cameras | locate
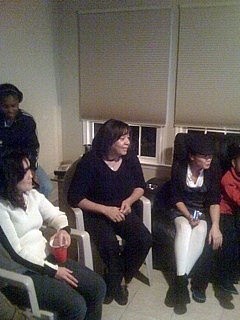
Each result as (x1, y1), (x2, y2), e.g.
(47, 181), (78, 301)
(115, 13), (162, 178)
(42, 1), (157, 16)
(193, 210), (202, 221)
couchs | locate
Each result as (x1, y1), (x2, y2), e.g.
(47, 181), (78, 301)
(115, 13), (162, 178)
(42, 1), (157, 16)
(144, 133), (240, 283)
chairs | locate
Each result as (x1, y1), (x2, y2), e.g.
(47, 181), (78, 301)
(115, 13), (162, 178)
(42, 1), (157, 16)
(62, 157), (154, 287)
(0, 226), (94, 320)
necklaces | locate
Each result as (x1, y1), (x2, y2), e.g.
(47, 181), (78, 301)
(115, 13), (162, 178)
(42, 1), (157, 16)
(103, 155), (122, 171)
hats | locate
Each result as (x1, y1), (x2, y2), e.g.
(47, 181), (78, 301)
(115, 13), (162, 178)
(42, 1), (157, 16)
(189, 134), (216, 155)
(227, 142), (240, 160)
(0, 83), (23, 103)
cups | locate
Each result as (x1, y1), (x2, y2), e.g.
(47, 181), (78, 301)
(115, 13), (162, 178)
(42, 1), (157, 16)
(49, 236), (70, 264)
(193, 210), (205, 227)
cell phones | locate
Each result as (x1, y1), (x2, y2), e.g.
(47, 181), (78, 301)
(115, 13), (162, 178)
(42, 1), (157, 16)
(32, 178), (40, 187)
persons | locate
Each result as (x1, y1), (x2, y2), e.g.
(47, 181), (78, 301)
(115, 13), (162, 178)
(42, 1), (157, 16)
(164, 135), (224, 316)
(67, 118), (152, 306)
(0, 150), (107, 320)
(0, 83), (53, 201)
(190, 145), (240, 303)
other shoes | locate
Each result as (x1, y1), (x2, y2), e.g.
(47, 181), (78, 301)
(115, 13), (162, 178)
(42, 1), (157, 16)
(174, 288), (189, 313)
(102, 277), (114, 304)
(114, 276), (128, 306)
(191, 284), (206, 303)
(164, 287), (175, 307)
(218, 281), (238, 294)
